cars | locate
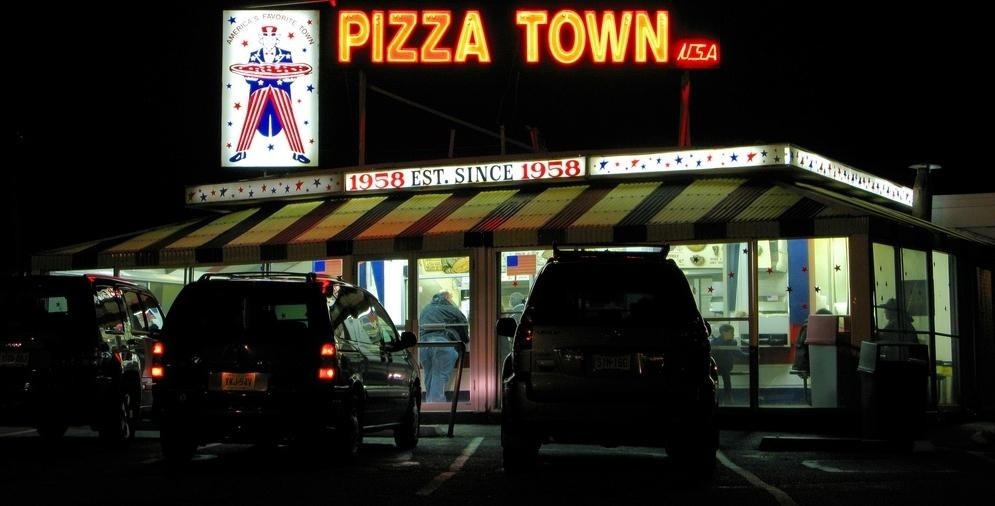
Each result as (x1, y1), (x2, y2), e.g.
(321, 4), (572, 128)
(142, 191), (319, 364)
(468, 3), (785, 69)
(150, 274), (422, 462)
(3, 273), (169, 443)
(493, 239), (722, 477)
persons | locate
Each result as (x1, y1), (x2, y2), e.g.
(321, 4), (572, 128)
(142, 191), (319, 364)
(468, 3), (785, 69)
(419, 291), (469, 403)
(793, 309), (850, 406)
(708, 324), (750, 405)
(507, 292), (525, 352)
(877, 296), (919, 357)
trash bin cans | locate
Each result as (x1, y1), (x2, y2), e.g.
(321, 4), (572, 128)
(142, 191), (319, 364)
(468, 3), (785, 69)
(803, 313), (929, 455)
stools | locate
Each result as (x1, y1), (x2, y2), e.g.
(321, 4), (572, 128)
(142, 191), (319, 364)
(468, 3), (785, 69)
(790, 370), (810, 403)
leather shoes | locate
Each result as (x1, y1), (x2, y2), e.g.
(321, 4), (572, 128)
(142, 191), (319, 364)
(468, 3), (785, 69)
(725, 391), (734, 405)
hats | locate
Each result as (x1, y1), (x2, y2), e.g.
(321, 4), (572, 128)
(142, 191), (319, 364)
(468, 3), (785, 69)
(510, 293), (524, 307)
(880, 298), (897, 309)
